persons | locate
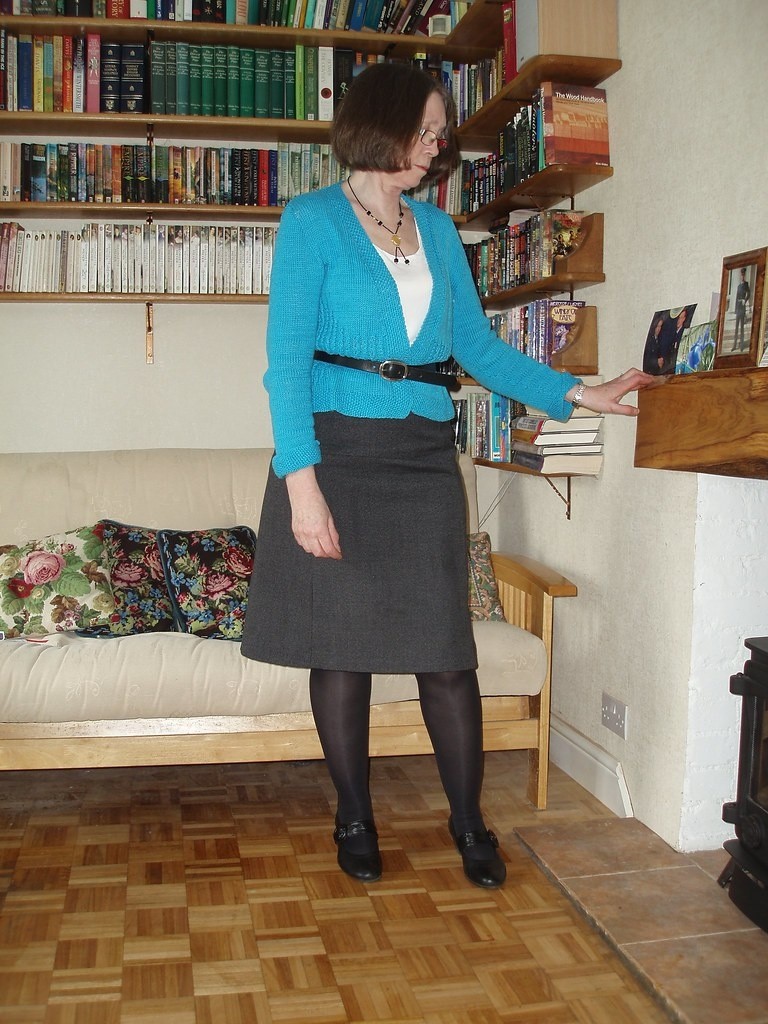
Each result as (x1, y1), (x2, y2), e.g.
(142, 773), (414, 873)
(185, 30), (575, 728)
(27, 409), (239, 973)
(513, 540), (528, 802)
(241, 62), (660, 890)
(646, 310), (687, 372)
(730, 268), (750, 351)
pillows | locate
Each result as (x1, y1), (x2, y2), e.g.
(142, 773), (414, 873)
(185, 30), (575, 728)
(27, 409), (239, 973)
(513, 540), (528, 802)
(0, 521), (121, 640)
(469, 532), (509, 622)
(154, 524), (257, 641)
(73, 519), (183, 640)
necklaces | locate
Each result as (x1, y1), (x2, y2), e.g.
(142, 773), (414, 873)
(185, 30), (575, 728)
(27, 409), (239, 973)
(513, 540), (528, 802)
(347, 175), (410, 266)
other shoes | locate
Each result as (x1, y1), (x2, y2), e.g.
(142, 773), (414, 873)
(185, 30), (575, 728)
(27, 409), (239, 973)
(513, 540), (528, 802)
(740, 347), (744, 351)
(731, 346), (736, 351)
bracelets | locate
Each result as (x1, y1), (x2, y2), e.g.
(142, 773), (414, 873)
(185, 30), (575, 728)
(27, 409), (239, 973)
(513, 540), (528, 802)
(571, 385), (587, 408)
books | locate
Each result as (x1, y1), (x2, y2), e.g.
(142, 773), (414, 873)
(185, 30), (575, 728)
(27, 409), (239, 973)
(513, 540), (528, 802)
(0, 0), (610, 294)
(439, 301), (605, 476)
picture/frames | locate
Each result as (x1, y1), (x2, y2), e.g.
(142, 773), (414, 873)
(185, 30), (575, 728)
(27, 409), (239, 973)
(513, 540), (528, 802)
(712, 246), (768, 370)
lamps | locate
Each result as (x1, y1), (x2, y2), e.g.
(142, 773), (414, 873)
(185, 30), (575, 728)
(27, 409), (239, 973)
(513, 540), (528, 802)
(428, 14), (451, 39)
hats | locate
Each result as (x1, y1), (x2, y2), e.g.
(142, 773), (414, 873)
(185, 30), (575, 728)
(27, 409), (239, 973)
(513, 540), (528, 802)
(741, 268), (746, 273)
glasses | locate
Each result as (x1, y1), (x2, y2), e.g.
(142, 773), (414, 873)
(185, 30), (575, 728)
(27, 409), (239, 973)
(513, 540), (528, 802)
(418, 127), (447, 150)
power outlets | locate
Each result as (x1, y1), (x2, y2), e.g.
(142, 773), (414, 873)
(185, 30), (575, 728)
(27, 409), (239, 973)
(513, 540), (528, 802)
(602, 692), (628, 741)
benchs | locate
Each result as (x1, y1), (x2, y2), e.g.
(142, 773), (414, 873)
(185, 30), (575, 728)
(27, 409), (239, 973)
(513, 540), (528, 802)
(0, 447), (578, 810)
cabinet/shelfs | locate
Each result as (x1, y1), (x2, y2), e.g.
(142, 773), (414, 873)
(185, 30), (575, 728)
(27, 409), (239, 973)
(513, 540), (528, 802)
(0, 0), (621, 520)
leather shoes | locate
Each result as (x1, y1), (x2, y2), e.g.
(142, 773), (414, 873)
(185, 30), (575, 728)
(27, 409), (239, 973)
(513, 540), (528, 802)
(333, 810), (380, 882)
(448, 813), (507, 889)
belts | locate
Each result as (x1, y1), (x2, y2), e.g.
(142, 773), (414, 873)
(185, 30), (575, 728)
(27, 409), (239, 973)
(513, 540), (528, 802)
(313, 350), (457, 388)
